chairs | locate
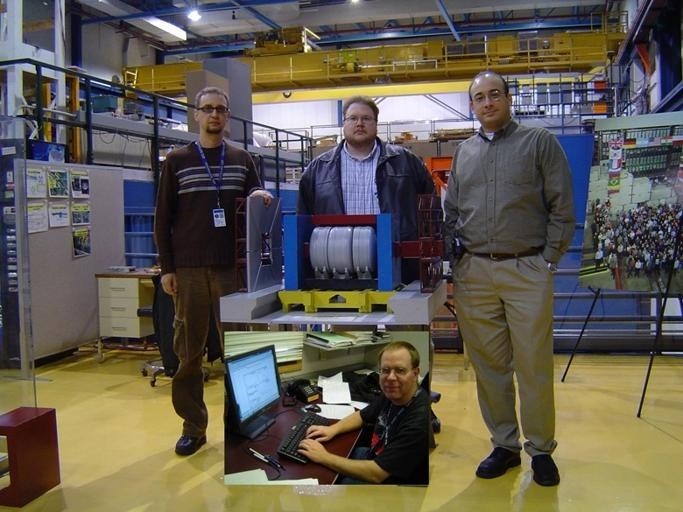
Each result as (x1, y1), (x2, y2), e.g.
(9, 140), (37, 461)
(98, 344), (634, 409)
(138, 276), (209, 387)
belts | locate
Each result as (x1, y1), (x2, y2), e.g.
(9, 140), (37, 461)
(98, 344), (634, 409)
(474, 250), (537, 261)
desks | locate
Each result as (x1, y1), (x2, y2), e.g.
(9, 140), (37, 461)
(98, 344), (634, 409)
(225, 381), (366, 484)
(95, 272), (163, 361)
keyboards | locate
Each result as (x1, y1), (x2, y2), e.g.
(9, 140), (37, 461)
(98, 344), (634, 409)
(277, 411), (330, 464)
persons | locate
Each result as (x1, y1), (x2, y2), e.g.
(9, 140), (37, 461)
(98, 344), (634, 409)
(298, 97), (445, 434)
(297, 341), (428, 485)
(154, 87), (275, 456)
(442, 71), (575, 485)
(590, 198), (683, 278)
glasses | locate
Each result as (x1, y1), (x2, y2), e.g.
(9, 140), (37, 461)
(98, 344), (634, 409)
(198, 104), (227, 115)
(377, 367), (411, 375)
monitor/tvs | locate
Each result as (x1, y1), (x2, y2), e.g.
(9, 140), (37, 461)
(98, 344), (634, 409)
(224, 344), (281, 441)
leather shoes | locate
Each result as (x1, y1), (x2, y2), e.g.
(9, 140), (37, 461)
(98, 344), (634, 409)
(530, 455), (560, 487)
(476, 448), (521, 478)
(175, 436), (206, 454)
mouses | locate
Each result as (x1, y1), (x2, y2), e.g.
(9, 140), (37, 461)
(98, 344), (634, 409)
(304, 404), (321, 413)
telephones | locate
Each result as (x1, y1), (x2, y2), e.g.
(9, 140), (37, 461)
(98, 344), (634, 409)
(287, 378), (319, 401)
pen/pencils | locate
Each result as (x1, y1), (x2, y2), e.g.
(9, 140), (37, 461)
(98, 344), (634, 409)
(248, 447), (287, 471)
